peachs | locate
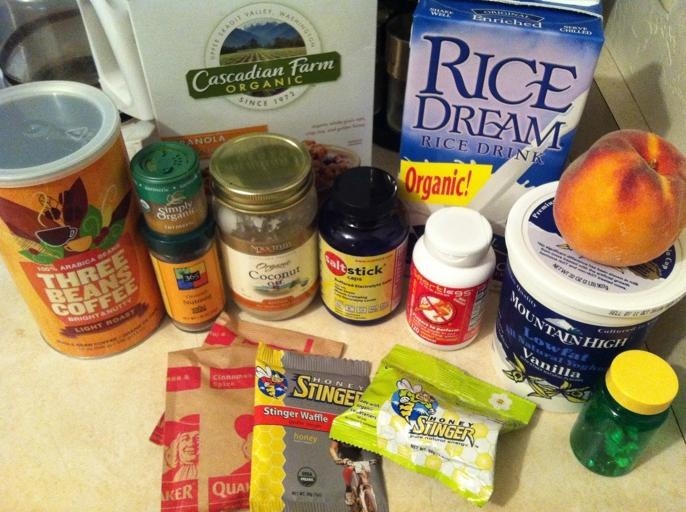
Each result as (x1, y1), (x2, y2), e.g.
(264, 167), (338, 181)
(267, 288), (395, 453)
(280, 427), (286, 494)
(553, 126), (686, 270)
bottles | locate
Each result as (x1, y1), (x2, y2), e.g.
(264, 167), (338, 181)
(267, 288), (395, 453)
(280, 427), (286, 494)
(316, 165), (411, 326)
(569, 349), (679, 478)
(404, 206), (496, 351)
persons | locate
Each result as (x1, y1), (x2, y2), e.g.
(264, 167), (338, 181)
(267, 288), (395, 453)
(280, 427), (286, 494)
(328, 440), (379, 512)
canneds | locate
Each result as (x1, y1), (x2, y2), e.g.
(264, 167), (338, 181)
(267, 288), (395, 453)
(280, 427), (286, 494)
(131, 140), (230, 332)
(208, 132), (320, 322)
